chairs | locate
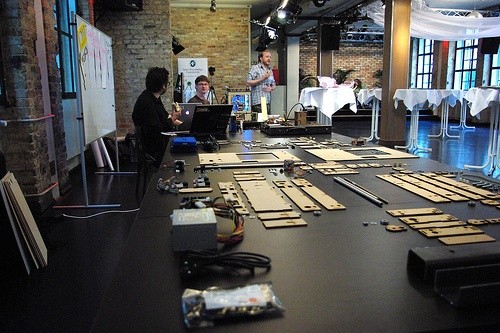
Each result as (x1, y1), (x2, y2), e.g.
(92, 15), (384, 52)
(125, 126), (162, 204)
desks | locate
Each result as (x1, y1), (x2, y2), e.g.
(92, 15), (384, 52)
(464, 86), (500, 177)
(89, 129), (500, 333)
(427, 89), (463, 139)
(393, 89), (436, 154)
(357, 88), (382, 142)
(450, 91), (481, 130)
(299, 86), (358, 127)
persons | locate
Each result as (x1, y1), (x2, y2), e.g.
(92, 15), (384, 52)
(352, 79), (367, 107)
(246, 50), (276, 114)
(132, 67), (182, 159)
(186, 74), (213, 105)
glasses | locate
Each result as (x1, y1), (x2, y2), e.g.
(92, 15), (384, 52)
(163, 81), (170, 85)
(197, 82), (208, 85)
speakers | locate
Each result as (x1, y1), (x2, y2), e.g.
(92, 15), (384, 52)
(321, 24), (340, 51)
(93, 0), (143, 11)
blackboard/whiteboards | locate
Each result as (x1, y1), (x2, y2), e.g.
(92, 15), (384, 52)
(76, 14), (116, 146)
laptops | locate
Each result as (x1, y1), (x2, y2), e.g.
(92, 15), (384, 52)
(175, 104), (234, 137)
